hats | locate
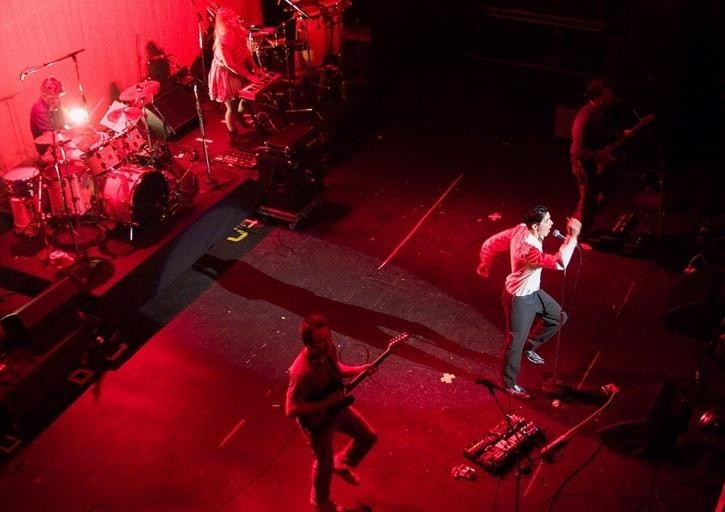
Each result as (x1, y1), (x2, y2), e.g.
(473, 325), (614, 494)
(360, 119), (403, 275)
(42, 78), (65, 98)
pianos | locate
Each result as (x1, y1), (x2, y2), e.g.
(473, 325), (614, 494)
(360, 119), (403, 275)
(239, 71), (283, 101)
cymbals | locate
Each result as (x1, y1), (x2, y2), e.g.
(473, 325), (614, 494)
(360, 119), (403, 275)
(35, 129), (72, 145)
(108, 107), (142, 122)
(119, 80), (160, 102)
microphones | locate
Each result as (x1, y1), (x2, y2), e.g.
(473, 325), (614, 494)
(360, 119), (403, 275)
(20, 72), (25, 80)
(474, 376), (511, 393)
(552, 229), (580, 249)
(48, 108), (55, 124)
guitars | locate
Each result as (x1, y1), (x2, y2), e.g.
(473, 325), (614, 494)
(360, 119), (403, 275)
(586, 115), (656, 175)
(297, 332), (409, 436)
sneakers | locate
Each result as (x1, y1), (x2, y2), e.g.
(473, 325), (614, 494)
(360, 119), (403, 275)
(318, 501), (338, 512)
(332, 466), (361, 486)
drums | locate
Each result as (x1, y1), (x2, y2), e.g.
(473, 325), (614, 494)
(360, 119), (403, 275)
(318, 0), (344, 56)
(79, 140), (122, 177)
(110, 124), (145, 161)
(295, 4), (327, 68)
(41, 160), (93, 218)
(97, 160), (169, 233)
(3, 166), (41, 199)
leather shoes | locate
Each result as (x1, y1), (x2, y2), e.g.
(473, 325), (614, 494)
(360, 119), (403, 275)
(524, 349), (545, 364)
(505, 384), (530, 399)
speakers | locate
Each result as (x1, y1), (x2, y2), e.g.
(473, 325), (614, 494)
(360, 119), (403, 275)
(662, 270), (721, 339)
(596, 382), (683, 458)
(140, 84), (205, 139)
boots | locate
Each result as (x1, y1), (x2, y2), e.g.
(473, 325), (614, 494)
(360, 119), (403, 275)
(228, 129), (250, 145)
(236, 112), (251, 127)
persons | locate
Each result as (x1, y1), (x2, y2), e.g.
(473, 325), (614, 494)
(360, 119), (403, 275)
(29, 77), (65, 156)
(207, 4), (265, 145)
(569, 78), (616, 250)
(147, 42), (171, 81)
(284, 314), (379, 512)
(476, 204), (582, 399)
